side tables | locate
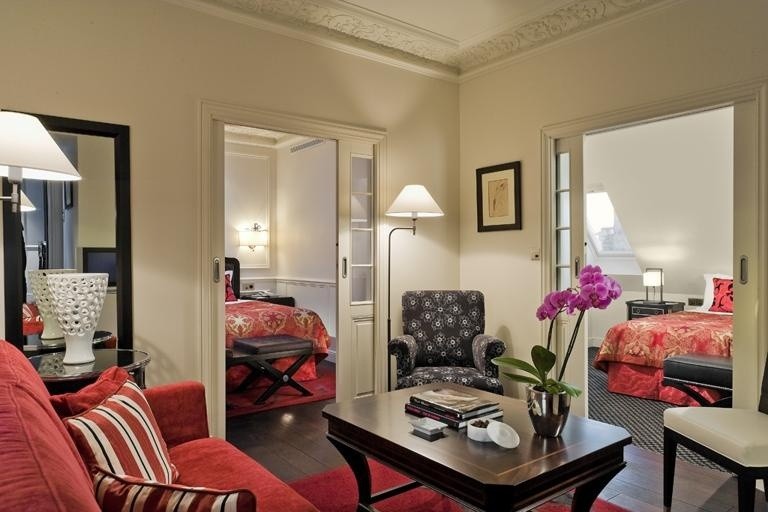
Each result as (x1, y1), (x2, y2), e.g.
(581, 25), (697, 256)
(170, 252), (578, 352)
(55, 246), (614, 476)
(28, 349), (151, 389)
(23, 331), (112, 357)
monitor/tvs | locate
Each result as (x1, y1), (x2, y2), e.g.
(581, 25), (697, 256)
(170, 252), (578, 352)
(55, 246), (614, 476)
(82, 247), (117, 293)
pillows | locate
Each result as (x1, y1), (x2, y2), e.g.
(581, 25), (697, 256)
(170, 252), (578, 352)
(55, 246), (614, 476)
(702, 273), (734, 315)
(49, 366), (256, 512)
(225, 270), (237, 302)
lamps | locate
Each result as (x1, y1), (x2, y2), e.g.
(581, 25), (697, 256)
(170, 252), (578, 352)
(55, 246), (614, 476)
(13, 184), (36, 212)
(643, 268), (665, 304)
(385, 185), (445, 392)
(0, 111), (82, 213)
(238, 223), (269, 257)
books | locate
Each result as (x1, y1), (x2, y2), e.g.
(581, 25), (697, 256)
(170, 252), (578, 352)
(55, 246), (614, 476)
(403, 401), (505, 431)
(410, 388), (501, 420)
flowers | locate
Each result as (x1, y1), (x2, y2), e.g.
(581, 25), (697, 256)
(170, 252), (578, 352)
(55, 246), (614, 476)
(491, 264), (623, 399)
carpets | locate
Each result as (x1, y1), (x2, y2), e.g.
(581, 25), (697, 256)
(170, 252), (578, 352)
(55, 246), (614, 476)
(288, 459), (632, 512)
(588, 346), (730, 473)
(226, 359), (336, 418)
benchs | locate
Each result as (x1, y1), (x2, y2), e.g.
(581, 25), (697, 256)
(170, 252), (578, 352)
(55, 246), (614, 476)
(661, 353), (733, 406)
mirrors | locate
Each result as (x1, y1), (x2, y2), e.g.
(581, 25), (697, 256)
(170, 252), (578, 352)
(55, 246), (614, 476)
(4, 110), (133, 370)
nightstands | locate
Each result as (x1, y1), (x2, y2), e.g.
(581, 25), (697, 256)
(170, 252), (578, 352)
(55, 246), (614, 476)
(243, 292), (295, 307)
(625, 300), (685, 320)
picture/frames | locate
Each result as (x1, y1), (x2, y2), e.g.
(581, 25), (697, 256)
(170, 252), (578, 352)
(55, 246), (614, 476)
(476, 161), (521, 232)
(63, 180), (73, 209)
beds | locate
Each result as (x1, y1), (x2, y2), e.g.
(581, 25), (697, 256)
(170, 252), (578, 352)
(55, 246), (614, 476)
(225, 257), (331, 393)
(606, 311), (733, 406)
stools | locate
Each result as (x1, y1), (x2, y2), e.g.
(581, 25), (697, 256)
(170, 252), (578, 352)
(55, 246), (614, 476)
(226, 335), (314, 404)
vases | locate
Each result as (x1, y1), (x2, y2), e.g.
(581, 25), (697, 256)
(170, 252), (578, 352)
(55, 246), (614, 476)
(526, 386), (571, 439)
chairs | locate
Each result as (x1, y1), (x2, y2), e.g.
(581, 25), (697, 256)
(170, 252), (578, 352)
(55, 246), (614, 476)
(388, 290), (506, 396)
(663, 353), (768, 512)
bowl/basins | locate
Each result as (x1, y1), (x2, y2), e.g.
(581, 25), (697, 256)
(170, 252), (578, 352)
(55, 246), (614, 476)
(467, 417), (520, 449)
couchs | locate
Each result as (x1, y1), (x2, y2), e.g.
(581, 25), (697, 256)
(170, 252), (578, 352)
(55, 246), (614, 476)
(0, 340), (320, 512)
